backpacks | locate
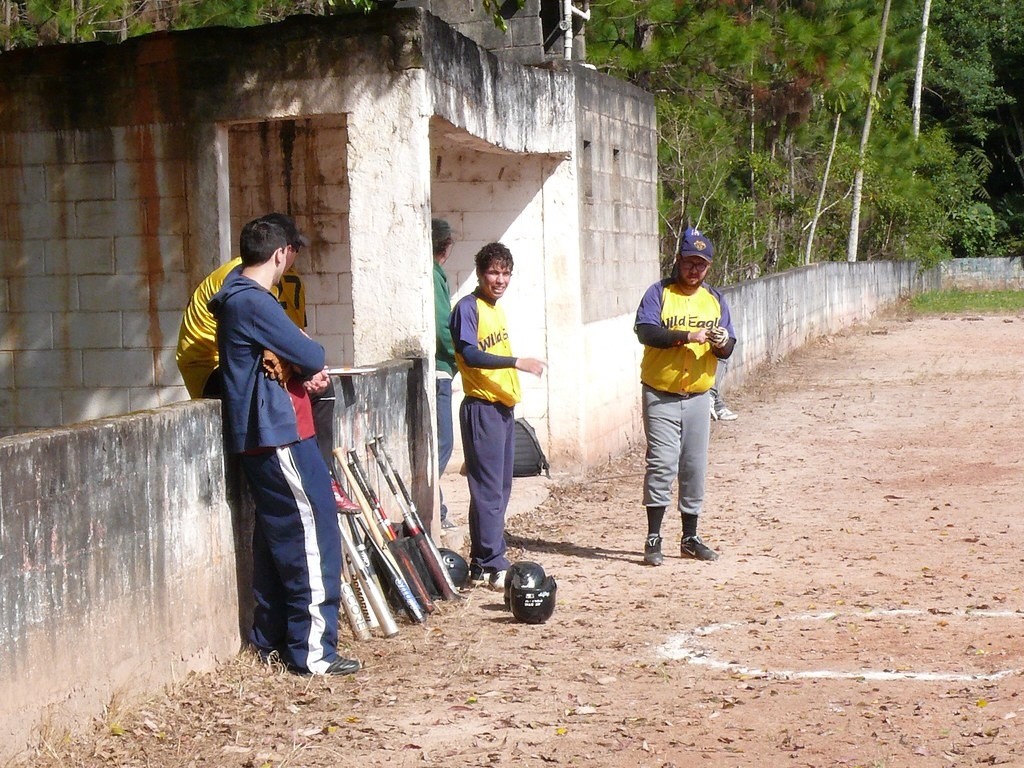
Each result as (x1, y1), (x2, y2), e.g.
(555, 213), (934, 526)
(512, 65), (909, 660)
(514, 416), (552, 480)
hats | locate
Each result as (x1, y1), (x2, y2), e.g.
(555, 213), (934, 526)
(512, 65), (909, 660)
(684, 226), (704, 234)
(680, 235), (714, 264)
(432, 218), (458, 243)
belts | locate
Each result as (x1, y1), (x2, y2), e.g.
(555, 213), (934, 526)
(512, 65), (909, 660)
(640, 381), (702, 399)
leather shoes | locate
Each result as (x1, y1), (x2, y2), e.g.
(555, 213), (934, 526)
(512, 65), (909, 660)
(331, 480), (362, 515)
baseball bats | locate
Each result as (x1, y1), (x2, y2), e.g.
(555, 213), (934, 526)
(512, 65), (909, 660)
(323, 432), (457, 643)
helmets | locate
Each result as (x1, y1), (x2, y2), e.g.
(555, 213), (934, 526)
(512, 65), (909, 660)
(503, 561), (557, 625)
(438, 547), (470, 587)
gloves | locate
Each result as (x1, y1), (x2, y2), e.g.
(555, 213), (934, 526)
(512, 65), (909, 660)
(705, 326), (730, 348)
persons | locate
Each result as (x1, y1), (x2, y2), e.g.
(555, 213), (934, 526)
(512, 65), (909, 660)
(634, 228), (737, 567)
(175, 214), (364, 517)
(449, 242), (549, 592)
(209, 220), (362, 674)
(432, 217), (459, 530)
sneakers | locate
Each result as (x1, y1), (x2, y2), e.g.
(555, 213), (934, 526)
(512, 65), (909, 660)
(680, 537), (720, 561)
(258, 650), (283, 664)
(644, 537), (664, 567)
(471, 570), (506, 593)
(325, 654), (361, 676)
(710, 393), (739, 422)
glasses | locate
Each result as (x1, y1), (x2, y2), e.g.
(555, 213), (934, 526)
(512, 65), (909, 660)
(680, 258), (709, 272)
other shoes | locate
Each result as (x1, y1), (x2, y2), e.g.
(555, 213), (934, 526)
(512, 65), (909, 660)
(440, 518), (457, 538)
